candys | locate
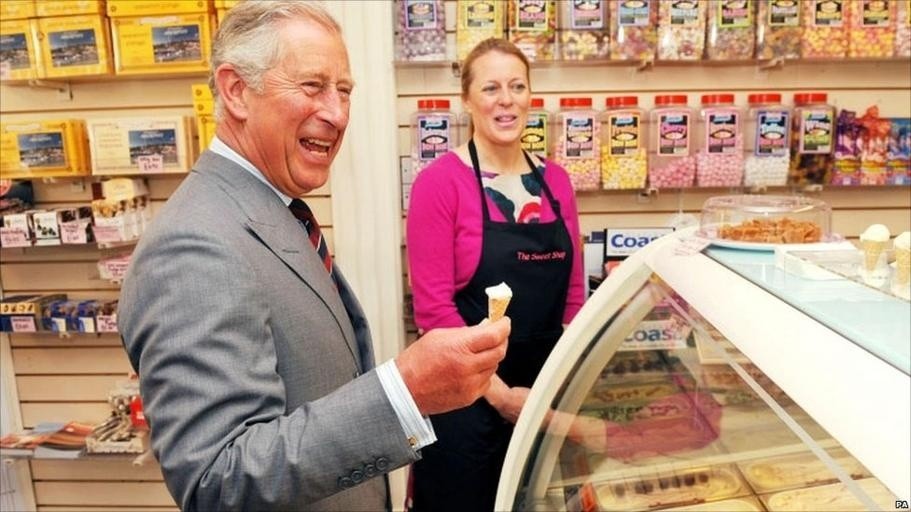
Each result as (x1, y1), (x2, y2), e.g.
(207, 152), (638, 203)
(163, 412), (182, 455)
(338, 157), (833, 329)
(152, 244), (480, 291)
(394, 0), (911, 65)
(412, 92), (911, 191)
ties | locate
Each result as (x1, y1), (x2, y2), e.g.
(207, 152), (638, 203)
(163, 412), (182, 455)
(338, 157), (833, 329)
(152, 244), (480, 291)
(289, 198), (339, 288)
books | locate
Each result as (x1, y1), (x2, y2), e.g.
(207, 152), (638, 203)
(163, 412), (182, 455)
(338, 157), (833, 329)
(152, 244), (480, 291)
(0, 420), (147, 459)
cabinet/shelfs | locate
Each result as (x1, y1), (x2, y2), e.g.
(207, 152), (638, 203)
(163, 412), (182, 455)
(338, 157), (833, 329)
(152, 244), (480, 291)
(391, 2), (911, 410)
(0, 2), (334, 511)
(492, 223), (911, 511)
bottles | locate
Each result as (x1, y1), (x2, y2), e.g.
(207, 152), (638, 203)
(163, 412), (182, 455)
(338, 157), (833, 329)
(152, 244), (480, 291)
(657, 1), (706, 58)
(455, 1), (505, 62)
(611, 1), (658, 60)
(394, 1), (448, 66)
(695, 93), (744, 188)
(804, 0), (849, 60)
(553, 97), (604, 188)
(601, 96), (648, 188)
(508, 3), (555, 63)
(755, 0), (803, 60)
(409, 101), (458, 177)
(558, 1), (613, 61)
(647, 96), (696, 188)
(849, 0), (897, 57)
(794, 93), (838, 186)
(895, 2), (911, 58)
(521, 99), (552, 158)
(741, 94), (793, 188)
(705, 0), (756, 61)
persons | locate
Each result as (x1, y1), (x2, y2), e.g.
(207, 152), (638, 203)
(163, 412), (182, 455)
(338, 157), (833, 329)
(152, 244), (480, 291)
(118, 1), (511, 512)
(511, 344), (795, 462)
(408, 39), (587, 511)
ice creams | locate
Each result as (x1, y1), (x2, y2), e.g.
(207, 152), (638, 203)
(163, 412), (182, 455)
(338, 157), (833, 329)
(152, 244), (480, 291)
(859, 224), (890, 273)
(484, 280), (514, 323)
(893, 230), (910, 291)
